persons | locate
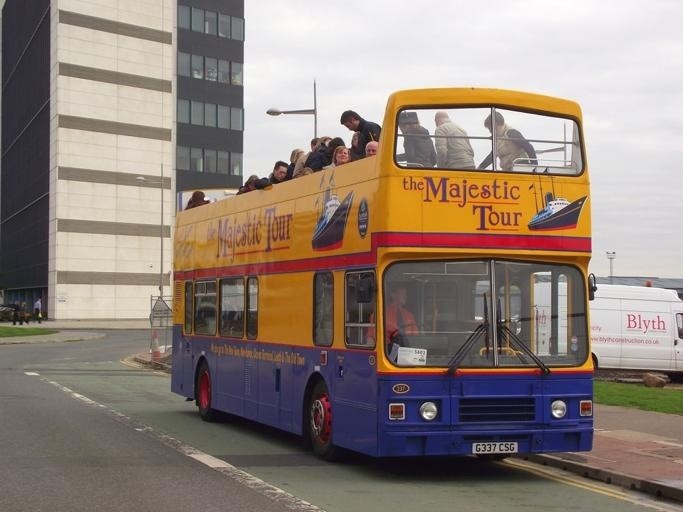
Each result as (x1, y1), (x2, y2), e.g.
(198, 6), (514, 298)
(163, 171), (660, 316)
(184, 199), (192, 210)
(12, 300), (20, 325)
(191, 191), (210, 208)
(32, 298), (42, 323)
(399, 111), (438, 167)
(19, 302), (28, 325)
(365, 284), (421, 345)
(434, 110), (475, 169)
(477, 111), (538, 172)
(236, 110), (381, 194)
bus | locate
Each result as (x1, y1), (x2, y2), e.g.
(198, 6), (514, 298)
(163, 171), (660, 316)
(166, 86), (595, 471)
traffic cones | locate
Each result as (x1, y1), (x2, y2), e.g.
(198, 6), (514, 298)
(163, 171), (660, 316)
(149, 328), (162, 361)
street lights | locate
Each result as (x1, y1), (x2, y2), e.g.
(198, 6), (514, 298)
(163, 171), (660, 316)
(605, 251), (616, 284)
(266, 79), (317, 140)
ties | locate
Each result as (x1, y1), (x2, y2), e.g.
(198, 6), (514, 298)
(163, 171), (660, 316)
(396, 308), (406, 337)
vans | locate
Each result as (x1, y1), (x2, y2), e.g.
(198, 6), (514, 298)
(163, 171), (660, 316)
(526, 281), (682, 381)
